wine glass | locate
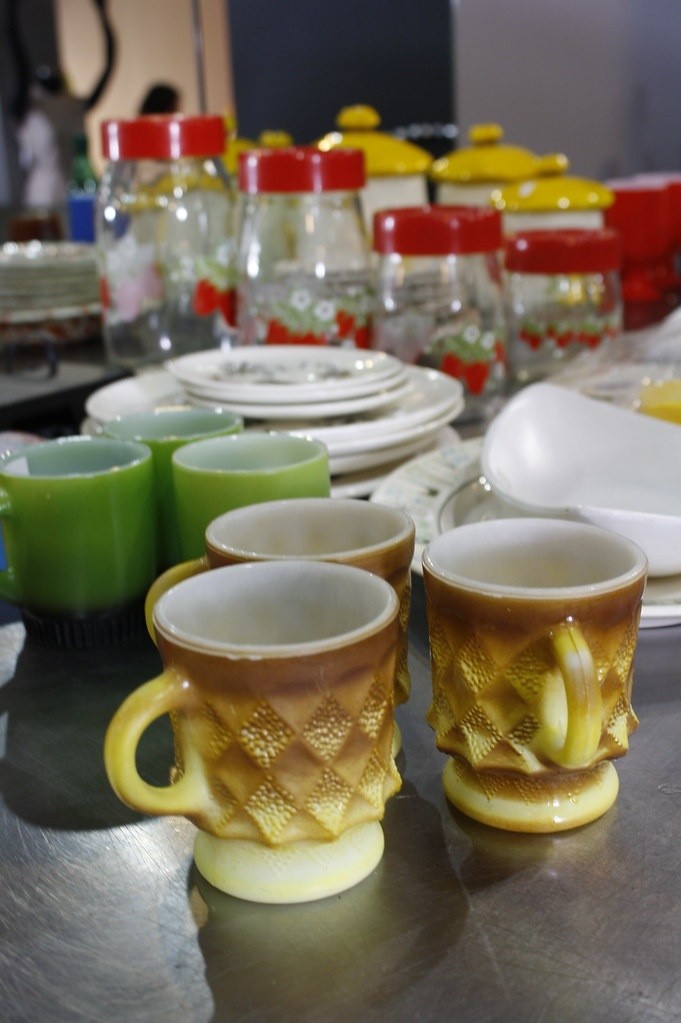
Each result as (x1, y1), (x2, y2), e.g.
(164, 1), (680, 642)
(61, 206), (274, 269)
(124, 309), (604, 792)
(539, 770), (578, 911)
(610, 177), (681, 303)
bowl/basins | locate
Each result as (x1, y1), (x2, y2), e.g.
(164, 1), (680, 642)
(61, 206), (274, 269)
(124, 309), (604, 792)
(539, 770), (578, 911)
(479, 382), (677, 579)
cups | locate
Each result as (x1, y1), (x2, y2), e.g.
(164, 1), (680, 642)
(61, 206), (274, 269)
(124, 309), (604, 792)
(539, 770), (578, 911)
(491, 154), (611, 232)
(369, 208), (502, 424)
(431, 126), (533, 212)
(231, 147), (374, 351)
(0, 401), (336, 603)
(422, 517), (642, 833)
(503, 227), (628, 390)
(321, 103), (430, 212)
(103, 560), (408, 908)
(90, 110), (235, 374)
(140, 500), (416, 762)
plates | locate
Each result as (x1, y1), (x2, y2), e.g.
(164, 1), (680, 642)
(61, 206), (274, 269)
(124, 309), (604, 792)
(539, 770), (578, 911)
(3, 243), (97, 325)
(374, 437), (681, 636)
(80, 341), (465, 500)
